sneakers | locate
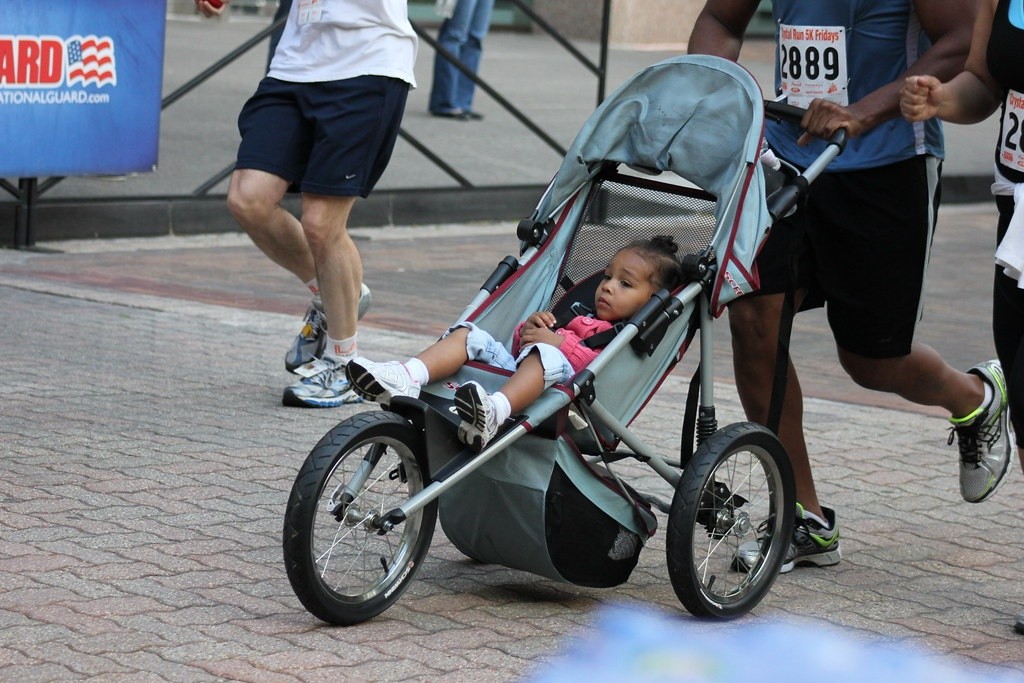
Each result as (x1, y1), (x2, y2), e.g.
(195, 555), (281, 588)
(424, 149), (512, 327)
(945, 359), (1015, 503)
(345, 356), (421, 411)
(454, 381), (499, 454)
(281, 349), (364, 408)
(731, 502), (840, 574)
(284, 282), (372, 375)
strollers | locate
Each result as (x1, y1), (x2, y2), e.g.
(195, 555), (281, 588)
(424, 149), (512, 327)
(282, 53), (847, 627)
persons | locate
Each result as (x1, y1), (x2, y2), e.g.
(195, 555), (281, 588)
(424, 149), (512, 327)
(345, 235), (683, 453)
(193, 0), (419, 407)
(900, 0), (1024, 472)
(688, 0), (1015, 573)
(430, 0), (495, 122)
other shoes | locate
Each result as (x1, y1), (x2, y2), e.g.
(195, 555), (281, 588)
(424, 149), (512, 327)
(432, 109), (482, 122)
